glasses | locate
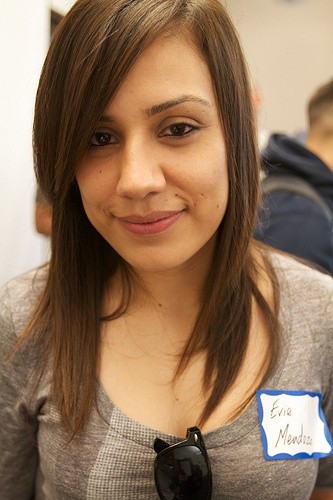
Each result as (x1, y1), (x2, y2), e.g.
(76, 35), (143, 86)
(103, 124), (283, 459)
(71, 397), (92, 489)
(146, 424), (215, 496)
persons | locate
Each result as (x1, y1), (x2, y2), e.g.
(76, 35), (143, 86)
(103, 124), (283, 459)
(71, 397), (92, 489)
(0, 0), (333, 500)
(253, 78), (333, 276)
